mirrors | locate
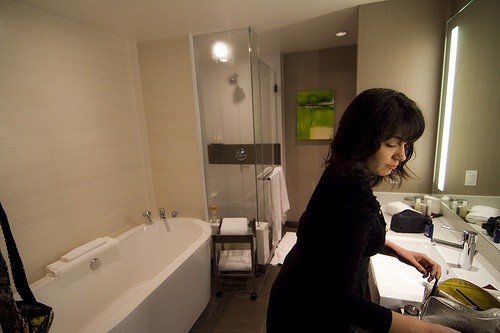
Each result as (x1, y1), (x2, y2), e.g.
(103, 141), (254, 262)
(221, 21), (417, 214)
(430, 0), (500, 252)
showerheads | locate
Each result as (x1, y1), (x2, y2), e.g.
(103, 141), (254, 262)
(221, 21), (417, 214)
(228, 76), (236, 84)
(143, 210), (154, 225)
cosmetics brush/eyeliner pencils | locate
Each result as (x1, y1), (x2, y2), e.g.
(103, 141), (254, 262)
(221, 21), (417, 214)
(456, 289), (484, 311)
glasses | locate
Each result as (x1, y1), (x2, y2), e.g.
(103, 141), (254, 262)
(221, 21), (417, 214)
(396, 304), (421, 316)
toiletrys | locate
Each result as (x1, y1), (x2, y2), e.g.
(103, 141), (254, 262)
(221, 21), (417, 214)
(424, 218), (434, 237)
(415, 194), (440, 215)
(441, 196), (467, 217)
(493, 226), (500, 243)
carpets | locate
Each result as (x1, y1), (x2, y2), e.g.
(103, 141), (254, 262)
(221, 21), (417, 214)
(270, 230), (299, 266)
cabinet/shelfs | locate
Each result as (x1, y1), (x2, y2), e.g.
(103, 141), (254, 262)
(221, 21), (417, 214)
(211, 217), (261, 300)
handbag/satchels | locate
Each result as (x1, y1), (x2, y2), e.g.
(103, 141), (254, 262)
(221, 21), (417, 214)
(418, 277), (500, 333)
(0, 202), (54, 333)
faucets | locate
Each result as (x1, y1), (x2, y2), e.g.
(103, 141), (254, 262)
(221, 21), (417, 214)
(433, 225), (479, 270)
(159, 208), (166, 220)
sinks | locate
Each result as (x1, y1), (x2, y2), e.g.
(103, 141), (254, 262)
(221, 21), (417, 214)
(371, 237), (460, 296)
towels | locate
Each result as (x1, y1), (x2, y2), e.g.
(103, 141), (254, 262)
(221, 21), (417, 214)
(465, 205), (500, 224)
(218, 250), (252, 271)
(270, 232), (297, 266)
(384, 202), (423, 215)
(268, 167), (290, 249)
(221, 217), (249, 235)
(45, 236), (119, 277)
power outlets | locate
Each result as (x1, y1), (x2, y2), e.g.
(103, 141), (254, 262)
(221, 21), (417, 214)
(389, 173), (403, 184)
(465, 170), (478, 185)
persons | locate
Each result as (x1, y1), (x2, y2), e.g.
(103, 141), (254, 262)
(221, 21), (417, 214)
(265, 86), (460, 333)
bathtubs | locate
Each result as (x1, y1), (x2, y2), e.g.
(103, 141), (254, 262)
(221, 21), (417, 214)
(13, 217), (212, 332)
